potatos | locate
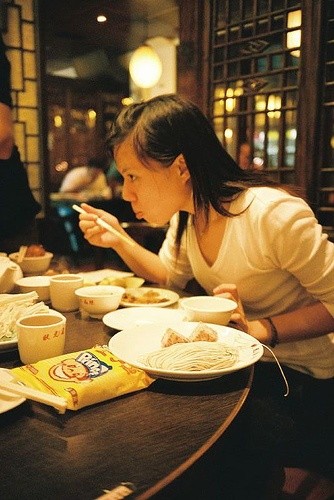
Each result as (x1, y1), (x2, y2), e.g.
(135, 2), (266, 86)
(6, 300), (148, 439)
(24, 244), (44, 257)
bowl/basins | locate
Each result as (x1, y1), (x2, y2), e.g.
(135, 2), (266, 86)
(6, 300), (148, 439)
(10, 251), (54, 275)
(75, 285), (126, 318)
(15, 275), (54, 306)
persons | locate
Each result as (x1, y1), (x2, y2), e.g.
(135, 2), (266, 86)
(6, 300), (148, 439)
(79, 94), (334, 378)
(59, 158), (112, 200)
(236, 141), (254, 169)
(0, 35), (42, 253)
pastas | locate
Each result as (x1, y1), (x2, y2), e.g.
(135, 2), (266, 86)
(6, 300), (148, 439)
(139, 340), (237, 372)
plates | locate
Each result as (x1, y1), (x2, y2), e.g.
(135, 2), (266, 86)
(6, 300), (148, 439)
(0, 294), (66, 354)
(118, 287), (181, 308)
(108, 320), (264, 383)
(102, 307), (185, 332)
(0, 366), (27, 414)
(80, 268), (137, 285)
(84, 276), (146, 291)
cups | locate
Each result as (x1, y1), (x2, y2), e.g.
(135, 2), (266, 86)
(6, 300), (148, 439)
(179, 295), (237, 328)
(15, 314), (68, 366)
(50, 273), (85, 313)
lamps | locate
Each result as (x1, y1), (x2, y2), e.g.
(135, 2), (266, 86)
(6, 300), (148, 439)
(128, 18), (162, 89)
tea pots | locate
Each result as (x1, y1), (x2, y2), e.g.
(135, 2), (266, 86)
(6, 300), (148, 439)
(0, 252), (24, 295)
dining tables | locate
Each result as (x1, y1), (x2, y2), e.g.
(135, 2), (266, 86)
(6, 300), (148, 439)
(0, 302), (255, 500)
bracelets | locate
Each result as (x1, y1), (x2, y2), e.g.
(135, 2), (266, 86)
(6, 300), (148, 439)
(264, 317), (278, 349)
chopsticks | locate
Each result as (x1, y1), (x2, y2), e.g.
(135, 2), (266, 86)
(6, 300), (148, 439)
(17, 245), (28, 263)
(0, 378), (68, 412)
(72, 203), (134, 246)
